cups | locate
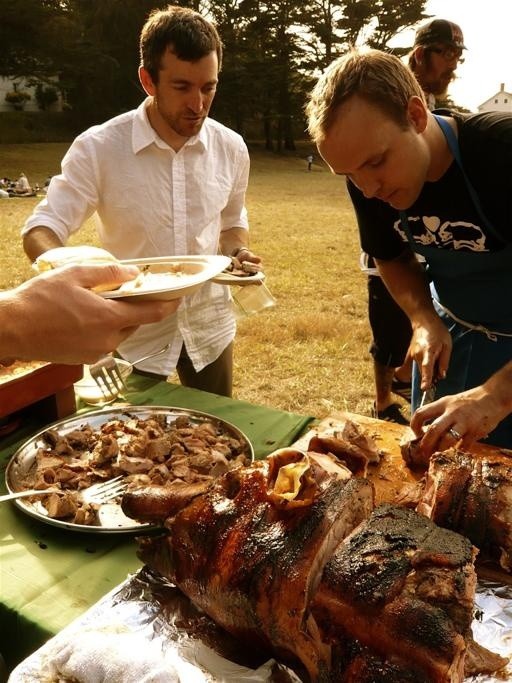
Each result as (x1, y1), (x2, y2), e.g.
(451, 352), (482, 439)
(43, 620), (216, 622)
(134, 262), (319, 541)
(231, 278), (279, 319)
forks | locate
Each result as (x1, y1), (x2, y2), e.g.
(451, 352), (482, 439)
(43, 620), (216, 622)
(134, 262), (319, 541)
(0, 473), (131, 505)
(90, 353), (129, 402)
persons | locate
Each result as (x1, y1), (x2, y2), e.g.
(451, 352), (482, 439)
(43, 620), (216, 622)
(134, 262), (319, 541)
(303, 48), (512, 455)
(19, 4), (262, 397)
(0, 264), (184, 366)
(366, 20), (467, 426)
(0, 172), (52, 197)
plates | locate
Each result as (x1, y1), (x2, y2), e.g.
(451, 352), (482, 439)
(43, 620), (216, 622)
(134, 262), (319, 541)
(4, 404), (254, 535)
(88, 253), (234, 303)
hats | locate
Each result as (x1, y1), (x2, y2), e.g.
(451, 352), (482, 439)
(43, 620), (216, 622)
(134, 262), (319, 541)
(414, 19), (467, 51)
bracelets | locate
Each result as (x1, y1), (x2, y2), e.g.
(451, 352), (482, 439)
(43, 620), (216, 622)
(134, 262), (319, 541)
(231, 246), (249, 256)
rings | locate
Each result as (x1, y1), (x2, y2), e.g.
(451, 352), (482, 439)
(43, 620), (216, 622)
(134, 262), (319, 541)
(449, 429), (461, 441)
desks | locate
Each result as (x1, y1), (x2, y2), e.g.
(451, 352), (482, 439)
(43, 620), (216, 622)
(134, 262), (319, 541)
(0, 371), (512, 683)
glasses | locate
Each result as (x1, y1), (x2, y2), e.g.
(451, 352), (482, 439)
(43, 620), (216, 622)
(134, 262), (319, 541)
(428, 46), (454, 62)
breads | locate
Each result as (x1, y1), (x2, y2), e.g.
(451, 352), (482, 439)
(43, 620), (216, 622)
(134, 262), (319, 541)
(37, 245), (123, 292)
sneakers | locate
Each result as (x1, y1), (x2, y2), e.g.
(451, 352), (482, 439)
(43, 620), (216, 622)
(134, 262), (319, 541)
(391, 374), (411, 405)
(374, 400), (411, 426)
